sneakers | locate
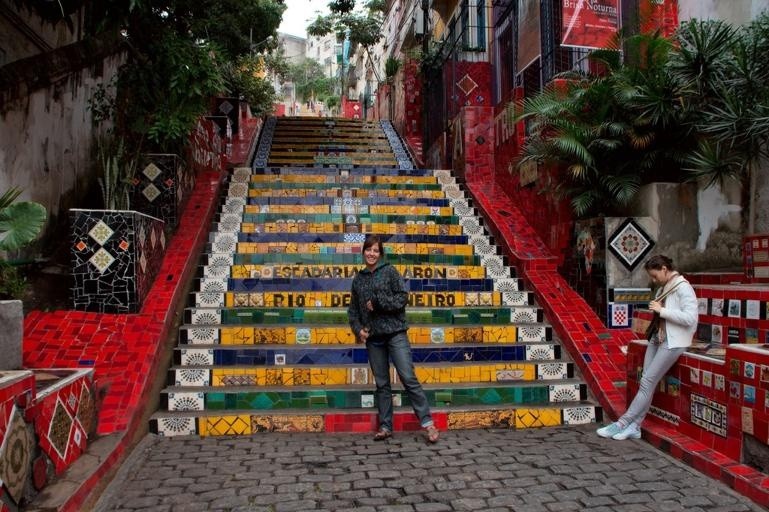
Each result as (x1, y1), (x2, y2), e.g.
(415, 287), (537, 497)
(375, 427), (392, 439)
(596, 421), (642, 440)
(423, 425), (440, 443)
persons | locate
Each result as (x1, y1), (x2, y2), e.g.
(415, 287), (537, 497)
(595, 254), (700, 440)
(347, 236), (441, 443)
(291, 100), (323, 117)
